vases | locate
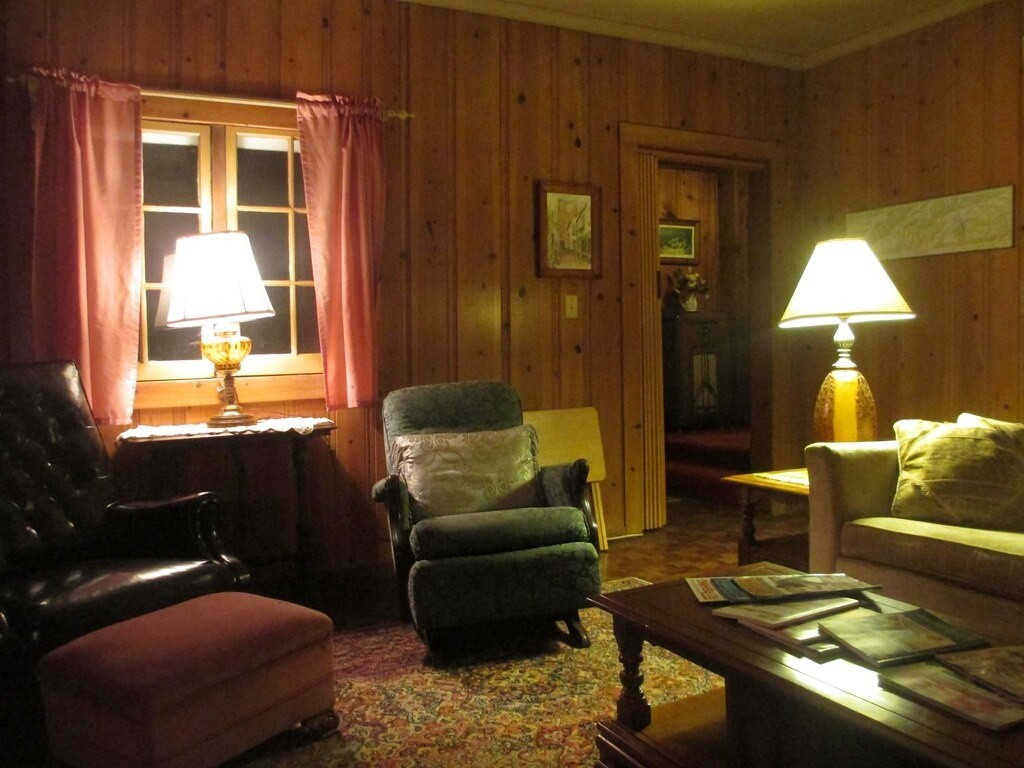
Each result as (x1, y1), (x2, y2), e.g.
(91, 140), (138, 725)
(679, 292), (697, 312)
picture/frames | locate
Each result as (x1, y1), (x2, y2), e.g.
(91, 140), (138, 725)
(658, 219), (702, 266)
(535, 178), (604, 280)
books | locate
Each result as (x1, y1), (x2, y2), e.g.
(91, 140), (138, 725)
(818, 606), (992, 668)
(686, 572), (909, 657)
(879, 644), (1024, 731)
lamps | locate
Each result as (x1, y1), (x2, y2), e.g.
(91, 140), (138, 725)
(777, 237), (916, 441)
(155, 230), (276, 428)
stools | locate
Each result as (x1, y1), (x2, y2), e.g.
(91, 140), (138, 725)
(37, 591), (340, 768)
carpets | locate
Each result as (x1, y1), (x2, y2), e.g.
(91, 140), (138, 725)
(212, 574), (723, 768)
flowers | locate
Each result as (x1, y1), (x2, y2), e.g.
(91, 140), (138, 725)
(672, 266), (710, 295)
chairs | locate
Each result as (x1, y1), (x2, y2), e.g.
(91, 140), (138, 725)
(372, 379), (601, 668)
(0, 358), (252, 674)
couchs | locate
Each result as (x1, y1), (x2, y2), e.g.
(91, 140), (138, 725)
(801, 442), (1024, 637)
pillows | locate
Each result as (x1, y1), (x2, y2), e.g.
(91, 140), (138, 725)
(891, 412), (1024, 531)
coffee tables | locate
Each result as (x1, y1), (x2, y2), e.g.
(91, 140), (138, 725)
(586, 562), (1024, 768)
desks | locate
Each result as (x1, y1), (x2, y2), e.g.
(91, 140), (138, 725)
(120, 415), (339, 607)
(519, 407), (610, 551)
(721, 467), (811, 577)
